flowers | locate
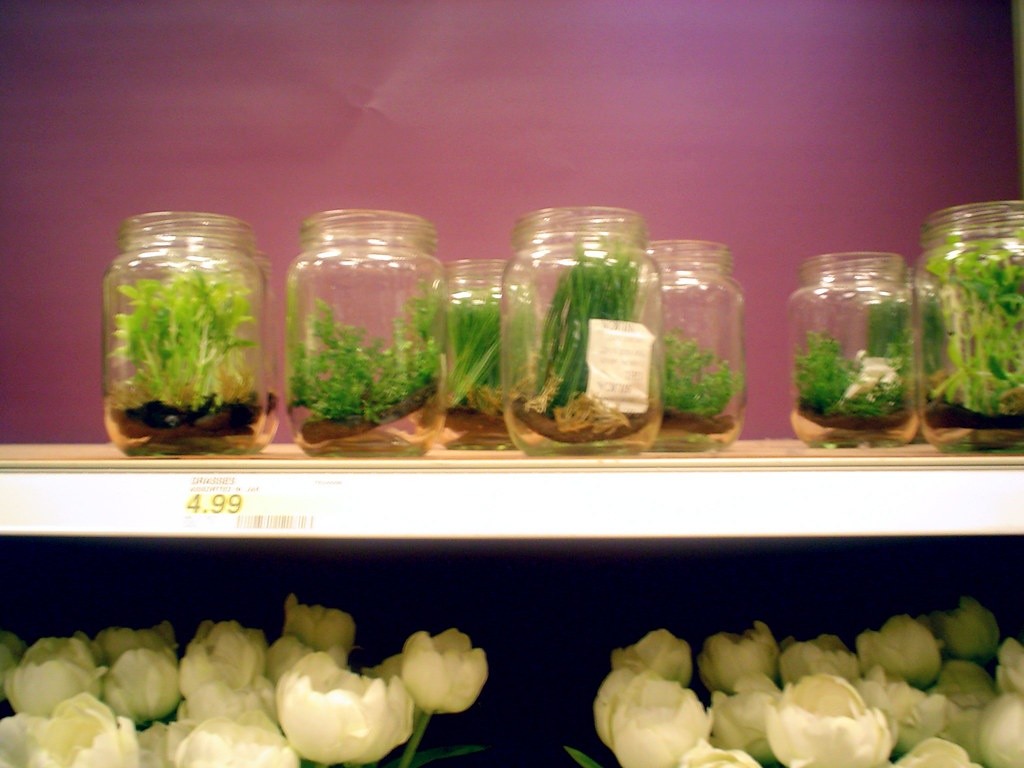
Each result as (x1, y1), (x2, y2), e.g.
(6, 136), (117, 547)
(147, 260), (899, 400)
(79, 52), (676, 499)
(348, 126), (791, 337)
(0, 592), (489, 768)
(563, 597), (1024, 768)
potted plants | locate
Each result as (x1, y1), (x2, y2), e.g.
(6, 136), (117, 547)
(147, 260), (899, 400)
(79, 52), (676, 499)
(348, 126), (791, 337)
(915, 198), (1023, 455)
(786, 251), (917, 449)
(444, 205), (749, 457)
(102, 215), (279, 458)
(281, 207), (444, 459)
